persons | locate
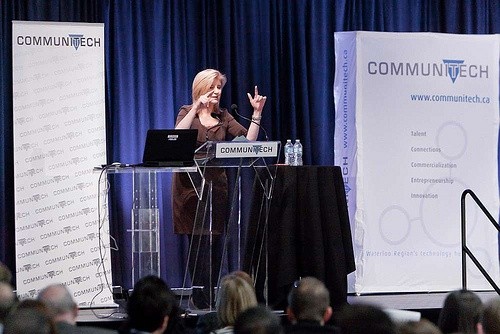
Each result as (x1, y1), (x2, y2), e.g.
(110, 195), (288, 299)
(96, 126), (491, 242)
(438, 289), (484, 333)
(36, 283), (119, 334)
(395, 319), (440, 334)
(286, 275), (333, 333)
(233, 307), (285, 333)
(332, 303), (398, 334)
(169, 68), (267, 312)
(125, 284), (171, 333)
(207, 274), (259, 333)
(134, 273), (167, 289)
(476, 294), (500, 334)
(5, 298), (57, 333)
(1, 262), (16, 333)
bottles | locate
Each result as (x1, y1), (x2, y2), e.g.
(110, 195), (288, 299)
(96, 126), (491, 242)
(285, 140), (293, 166)
(293, 140), (302, 166)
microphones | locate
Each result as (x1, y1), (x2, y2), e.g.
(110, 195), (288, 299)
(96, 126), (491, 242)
(231, 104), (269, 142)
(205, 112), (223, 141)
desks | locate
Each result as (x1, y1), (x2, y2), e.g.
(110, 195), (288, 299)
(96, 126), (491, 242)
(91, 165), (357, 312)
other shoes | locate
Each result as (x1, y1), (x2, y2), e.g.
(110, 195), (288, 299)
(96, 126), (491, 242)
(190, 297), (212, 311)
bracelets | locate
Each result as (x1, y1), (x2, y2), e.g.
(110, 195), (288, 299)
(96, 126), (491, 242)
(252, 115), (262, 121)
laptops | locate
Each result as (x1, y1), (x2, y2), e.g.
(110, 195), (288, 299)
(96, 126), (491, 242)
(121, 128), (199, 167)
